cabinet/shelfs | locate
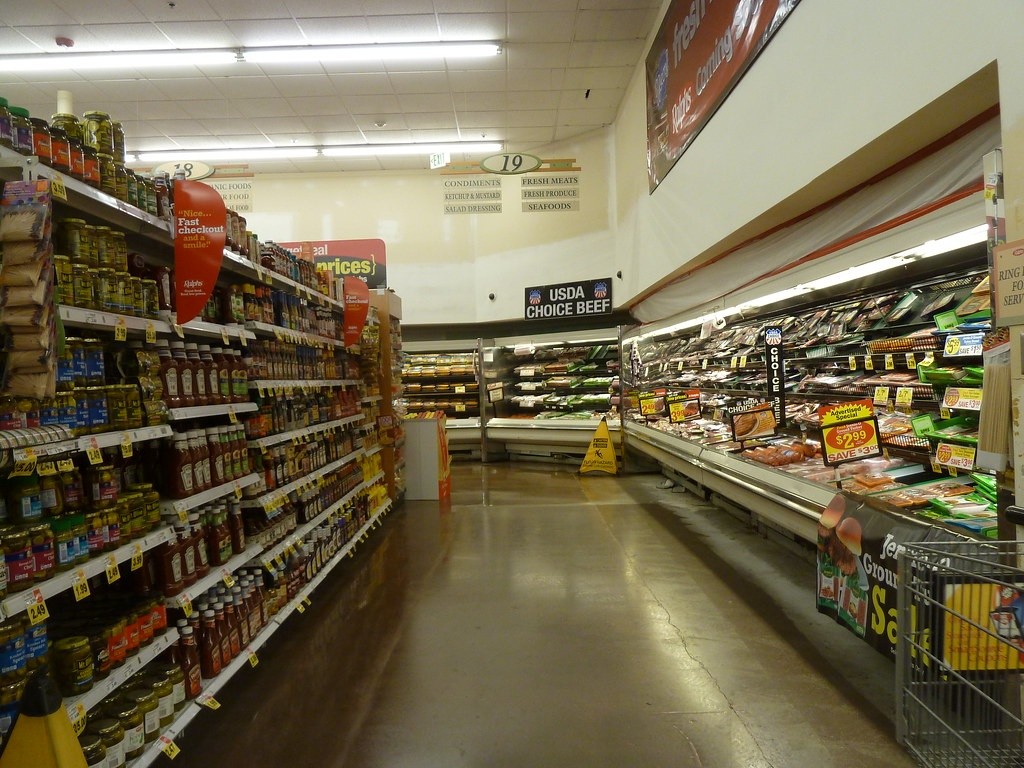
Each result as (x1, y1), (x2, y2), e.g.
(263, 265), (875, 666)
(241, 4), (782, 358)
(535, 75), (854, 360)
(404, 411), (452, 501)
(623, 209), (1004, 605)
(400, 325), (622, 473)
(0, 101), (407, 768)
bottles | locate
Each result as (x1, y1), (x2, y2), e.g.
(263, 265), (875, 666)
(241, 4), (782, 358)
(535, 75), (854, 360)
(265, 239), (319, 290)
(223, 208), (248, 258)
(247, 231), (262, 265)
(253, 337), (336, 379)
(242, 283), (274, 325)
(149, 423), (250, 495)
(276, 290), (344, 341)
(251, 388), (370, 612)
(129, 252), (241, 328)
(153, 496), (247, 596)
(144, 168), (187, 220)
(145, 339), (250, 406)
(168, 568), (269, 697)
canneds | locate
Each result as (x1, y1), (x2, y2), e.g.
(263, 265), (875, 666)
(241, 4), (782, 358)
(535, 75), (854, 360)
(0, 465), (161, 601)
(51, 219), (161, 319)
(0, 335), (144, 438)
(0, 96), (161, 218)
(0, 592), (186, 768)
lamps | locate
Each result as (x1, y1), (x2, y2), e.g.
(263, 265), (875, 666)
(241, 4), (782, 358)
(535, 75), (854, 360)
(1, 39), (506, 70)
(127, 139), (502, 163)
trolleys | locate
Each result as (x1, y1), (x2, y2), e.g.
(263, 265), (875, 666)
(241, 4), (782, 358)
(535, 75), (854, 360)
(891, 541), (1024, 768)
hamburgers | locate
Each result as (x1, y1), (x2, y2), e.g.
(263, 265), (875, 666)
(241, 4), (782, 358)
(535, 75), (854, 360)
(817, 495), (863, 577)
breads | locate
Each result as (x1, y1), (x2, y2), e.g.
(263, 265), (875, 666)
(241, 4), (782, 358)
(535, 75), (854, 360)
(742, 442), (824, 466)
